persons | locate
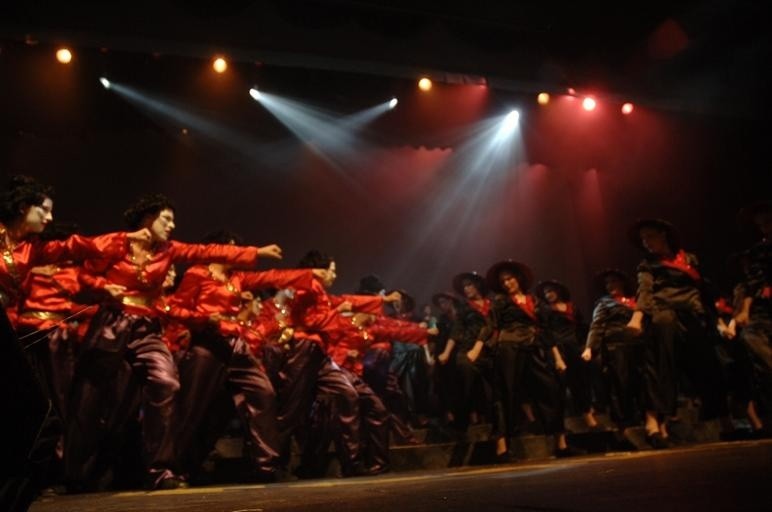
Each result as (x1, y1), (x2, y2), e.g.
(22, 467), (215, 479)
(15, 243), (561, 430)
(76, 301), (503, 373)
(0, 185), (772, 511)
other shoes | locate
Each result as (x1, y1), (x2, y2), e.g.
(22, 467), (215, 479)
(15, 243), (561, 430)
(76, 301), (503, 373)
(20, 454), (391, 504)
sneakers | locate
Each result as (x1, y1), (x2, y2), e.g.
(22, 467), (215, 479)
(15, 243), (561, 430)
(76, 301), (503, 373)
(486, 414), (766, 465)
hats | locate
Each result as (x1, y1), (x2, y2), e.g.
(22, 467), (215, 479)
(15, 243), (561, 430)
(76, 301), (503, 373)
(450, 270), (484, 296)
(628, 212), (689, 255)
(534, 280), (570, 302)
(484, 256), (531, 293)
(385, 285), (417, 316)
(592, 264), (636, 296)
(432, 289), (459, 316)
